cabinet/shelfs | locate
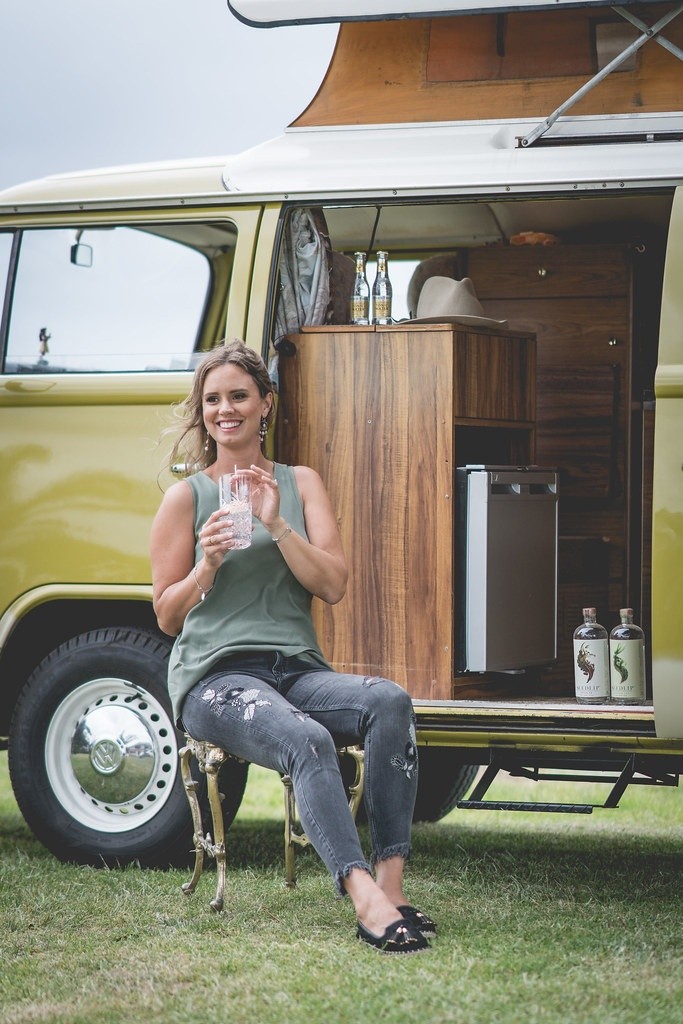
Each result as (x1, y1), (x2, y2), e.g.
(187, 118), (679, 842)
(277, 326), (539, 700)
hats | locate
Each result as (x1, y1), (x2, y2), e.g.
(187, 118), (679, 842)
(390, 276), (507, 326)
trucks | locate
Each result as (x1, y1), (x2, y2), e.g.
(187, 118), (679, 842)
(0, 0), (683, 868)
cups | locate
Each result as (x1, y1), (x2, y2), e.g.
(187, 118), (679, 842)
(218, 472), (252, 550)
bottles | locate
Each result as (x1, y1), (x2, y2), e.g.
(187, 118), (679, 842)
(610, 609), (646, 705)
(349, 252), (370, 325)
(372, 251), (393, 325)
(572, 607), (611, 705)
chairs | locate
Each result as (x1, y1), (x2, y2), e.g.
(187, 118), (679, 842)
(171, 459), (366, 915)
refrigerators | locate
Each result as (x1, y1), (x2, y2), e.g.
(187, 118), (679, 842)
(456, 464), (561, 673)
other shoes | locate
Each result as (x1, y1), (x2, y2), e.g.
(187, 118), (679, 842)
(396, 905), (435, 939)
(355, 921), (431, 955)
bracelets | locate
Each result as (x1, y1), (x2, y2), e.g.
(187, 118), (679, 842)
(272, 522), (292, 544)
(193, 561), (215, 601)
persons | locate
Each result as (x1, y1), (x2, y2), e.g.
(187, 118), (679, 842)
(149, 334), (437, 953)
